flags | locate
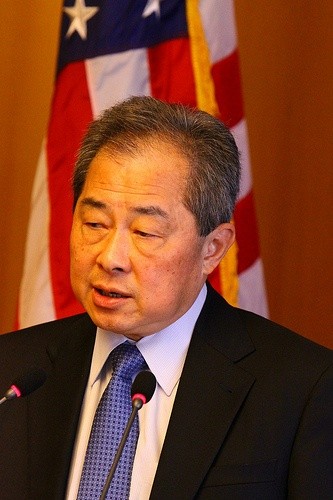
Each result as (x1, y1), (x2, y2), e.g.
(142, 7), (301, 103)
(13, 0), (269, 331)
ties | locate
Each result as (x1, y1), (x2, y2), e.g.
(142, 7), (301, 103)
(76, 345), (148, 498)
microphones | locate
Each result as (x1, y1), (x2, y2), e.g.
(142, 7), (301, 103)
(0, 368), (46, 405)
(99, 370), (156, 500)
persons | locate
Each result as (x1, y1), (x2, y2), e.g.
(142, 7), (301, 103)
(0, 97), (333, 500)
